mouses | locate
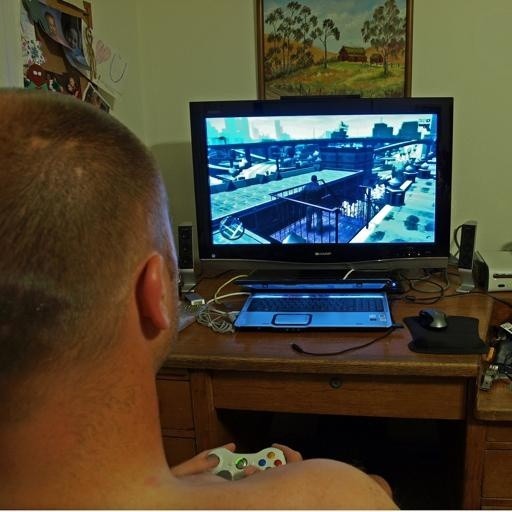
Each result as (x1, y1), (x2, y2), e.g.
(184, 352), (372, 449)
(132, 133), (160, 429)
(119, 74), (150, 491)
(418, 309), (449, 330)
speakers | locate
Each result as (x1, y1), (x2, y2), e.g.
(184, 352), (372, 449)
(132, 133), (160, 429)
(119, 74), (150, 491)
(456, 220), (479, 294)
(178, 221), (194, 294)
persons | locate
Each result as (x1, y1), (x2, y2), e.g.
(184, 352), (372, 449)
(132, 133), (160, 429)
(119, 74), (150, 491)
(1, 86), (397, 507)
(305, 176), (322, 234)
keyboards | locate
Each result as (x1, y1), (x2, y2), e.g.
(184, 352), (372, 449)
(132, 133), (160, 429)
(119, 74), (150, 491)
(232, 291), (394, 333)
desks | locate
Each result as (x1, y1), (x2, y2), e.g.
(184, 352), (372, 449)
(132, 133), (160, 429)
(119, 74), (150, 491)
(141, 263), (511, 508)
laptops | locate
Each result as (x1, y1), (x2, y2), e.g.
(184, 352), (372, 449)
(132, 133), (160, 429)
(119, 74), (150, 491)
(187, 98), (453, 295)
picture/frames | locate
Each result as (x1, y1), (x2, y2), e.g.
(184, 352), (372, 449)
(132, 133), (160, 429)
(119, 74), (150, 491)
(255, 1), (414, 100)
